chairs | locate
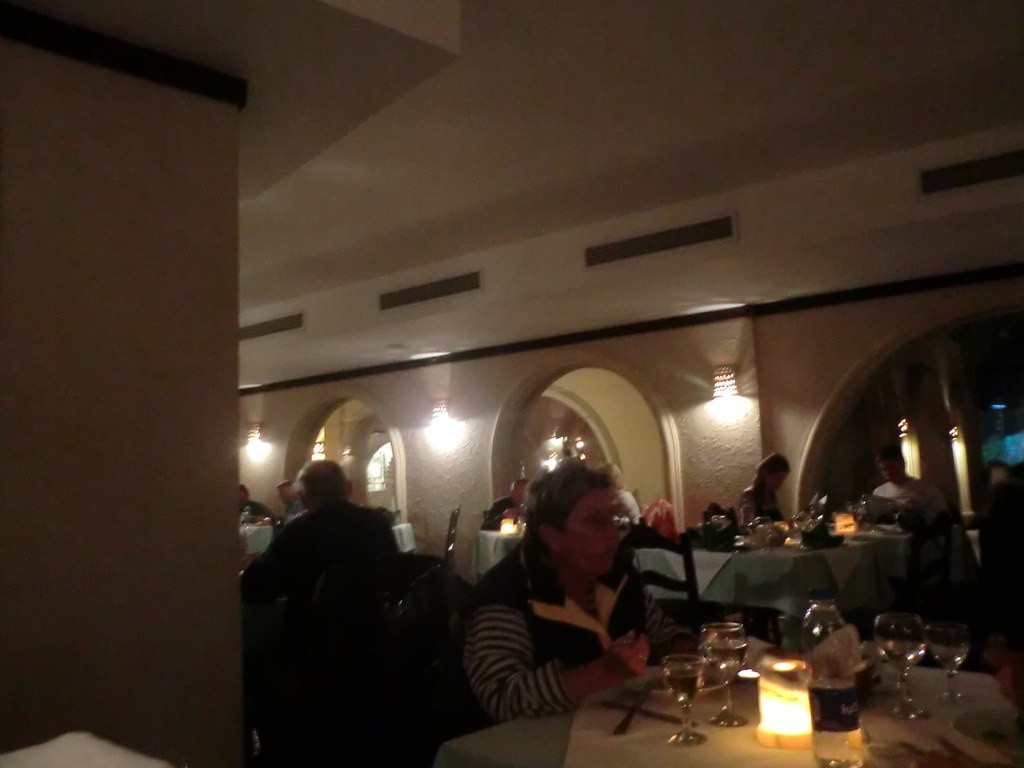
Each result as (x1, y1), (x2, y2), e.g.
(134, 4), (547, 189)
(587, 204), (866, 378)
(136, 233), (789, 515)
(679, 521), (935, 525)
(615, 524), (706, 642)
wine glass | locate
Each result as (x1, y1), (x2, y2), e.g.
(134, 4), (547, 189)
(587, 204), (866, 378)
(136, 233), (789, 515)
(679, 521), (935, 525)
(710, 494), (908, 553)
(925, 621), (972, 706)
(873, 612), (928, 720)
(661, 654), (708, 747)
(697, 623), (748, 727)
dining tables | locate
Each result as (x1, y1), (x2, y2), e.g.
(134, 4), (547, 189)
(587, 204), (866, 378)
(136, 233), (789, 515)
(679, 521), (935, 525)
(476, 528), (525, 582)
(431, 641), (1023, 768)
(240, 524), (274, 559)
(628, 536), (890, 645)
(851, 528), (983, 614)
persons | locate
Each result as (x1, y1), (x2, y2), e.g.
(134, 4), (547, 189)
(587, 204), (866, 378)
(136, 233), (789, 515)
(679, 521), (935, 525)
(238, 485), (272, 520)
(869, 444), (931, 514)
(241, 460), (406, 681)
(461, 460), (700, 721)
(277, 481), (308, 515)
(735, 454), (803, 529)
(480, 480), (531, 530)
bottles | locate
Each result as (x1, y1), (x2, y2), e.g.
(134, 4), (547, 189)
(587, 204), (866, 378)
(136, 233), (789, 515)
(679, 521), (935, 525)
(800, 587), (865, 768)
